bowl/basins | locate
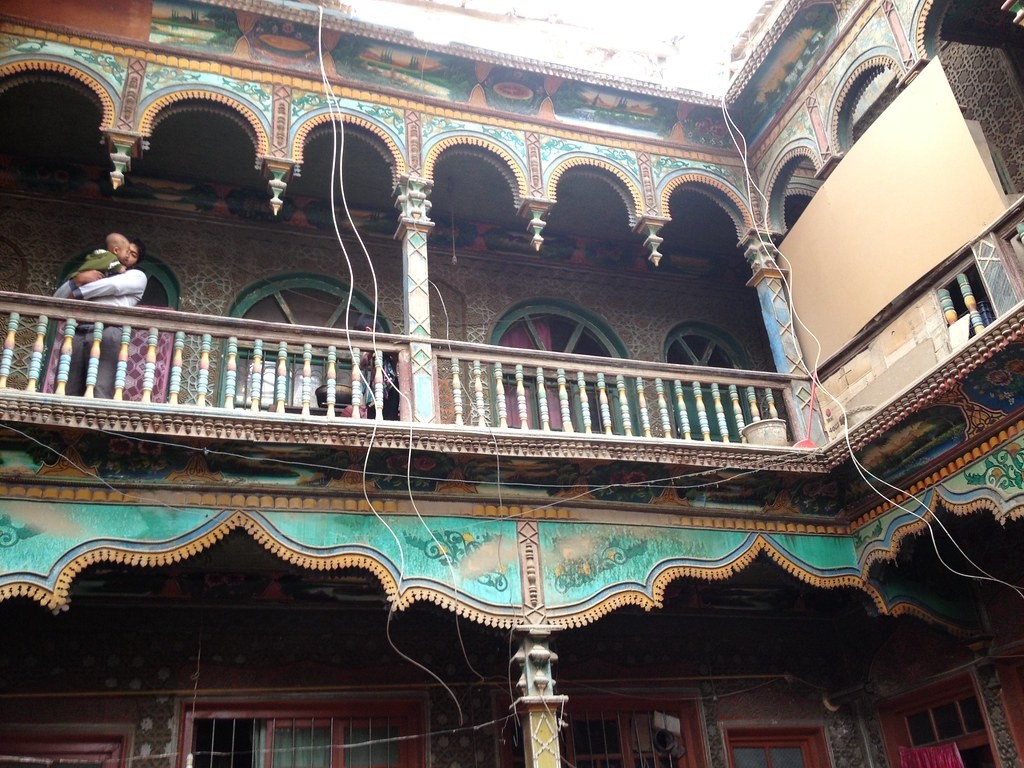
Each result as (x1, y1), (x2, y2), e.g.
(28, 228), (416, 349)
(315, 384), (352, 408)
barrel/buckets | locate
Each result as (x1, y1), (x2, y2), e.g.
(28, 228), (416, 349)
(743, 419), (787, 446)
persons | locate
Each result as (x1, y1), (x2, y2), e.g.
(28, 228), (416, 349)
(354, 317), (400, 421)
(52, 238), (147, 400)
(68, 232), (130, 279)
